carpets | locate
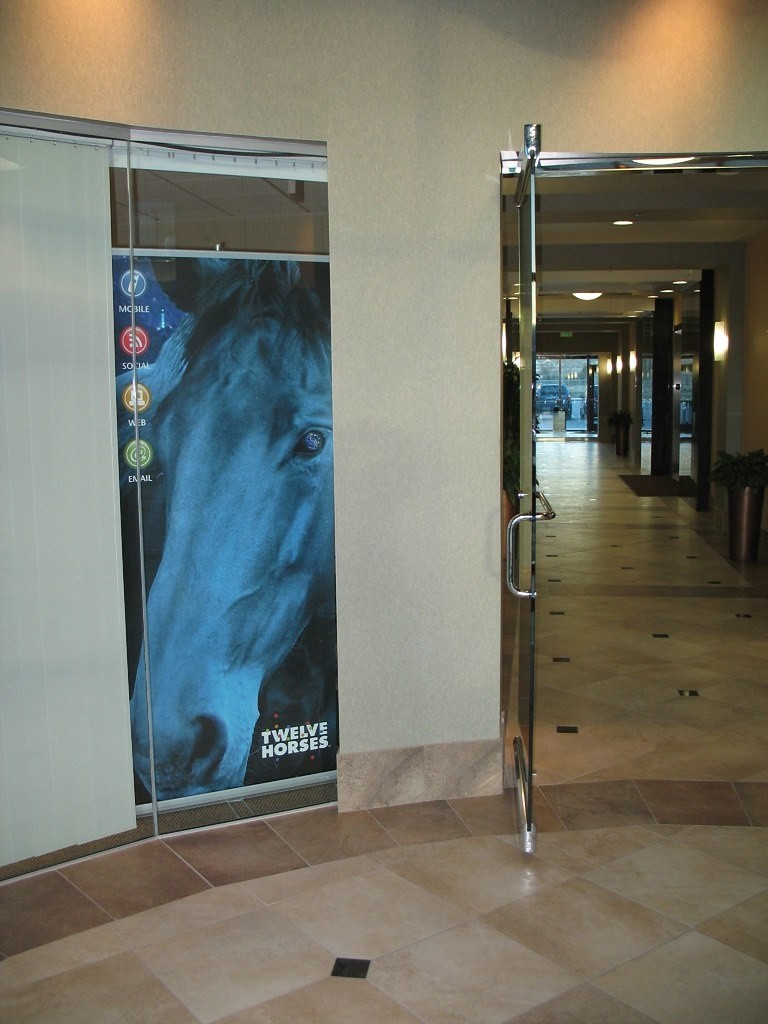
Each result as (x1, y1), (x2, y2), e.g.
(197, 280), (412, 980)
(618, 475), (700, 498)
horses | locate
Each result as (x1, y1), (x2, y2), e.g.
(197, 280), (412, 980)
(112, 259), (337, 799)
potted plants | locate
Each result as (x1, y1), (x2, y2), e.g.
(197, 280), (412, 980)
(606, 408), (632, 456)
(708, 449), (768, 562)
(503, 359), (539, 562)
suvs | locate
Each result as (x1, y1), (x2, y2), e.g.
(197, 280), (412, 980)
(580, 384), (599, 419)
(534, 378), (573, 419)
